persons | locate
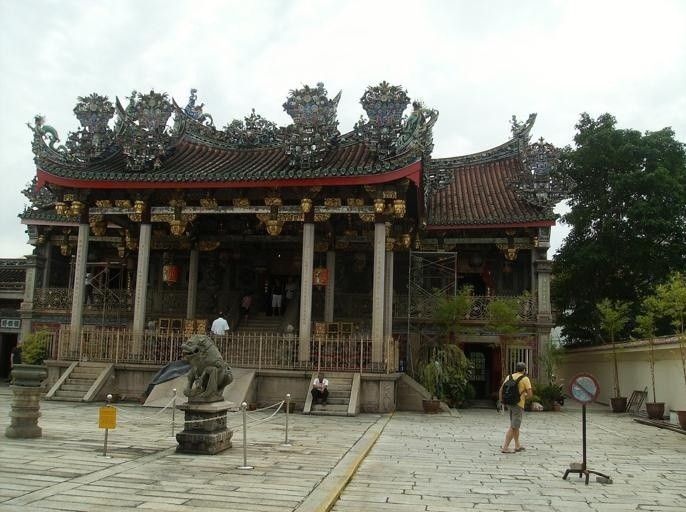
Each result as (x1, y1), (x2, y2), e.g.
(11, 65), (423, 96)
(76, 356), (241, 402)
(84, 268), (97, 305)
(210, 312), (230, 363)
(9, 339), (23, 383)
(498, 361), (534, 453)
(310, 370), (330, 405)
(240, 274), (297, 327)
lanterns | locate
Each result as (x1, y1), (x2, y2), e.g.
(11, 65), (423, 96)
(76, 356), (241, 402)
(313, 265), (329, 291)
(162, 264), (177, 287)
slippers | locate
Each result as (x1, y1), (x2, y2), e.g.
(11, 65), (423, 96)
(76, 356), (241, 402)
(516, 447), (525, 452)
(502, 448), (515, 453)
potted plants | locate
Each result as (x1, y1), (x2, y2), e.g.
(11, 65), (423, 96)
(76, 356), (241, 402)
(654, 268), (686, 431)
(633, 295), (666, 420)
(544, 385), (563, 411)
(596, 297), (634, 413)
(420, 357), (443, 414)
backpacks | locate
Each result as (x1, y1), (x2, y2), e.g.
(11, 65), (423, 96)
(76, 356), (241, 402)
(501, 374), (527, 405)
